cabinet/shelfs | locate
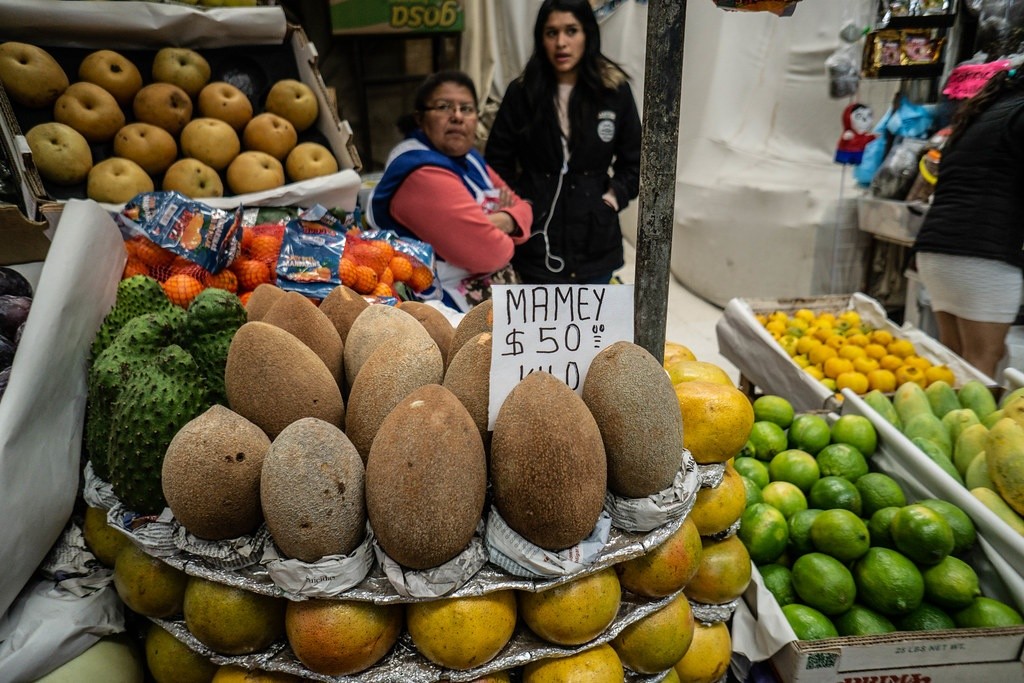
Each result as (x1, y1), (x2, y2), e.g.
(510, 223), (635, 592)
(863, 0), (959, 79)
(349, 34), (459, 160)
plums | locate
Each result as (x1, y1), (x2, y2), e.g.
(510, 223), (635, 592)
(0, 266), (34, 405)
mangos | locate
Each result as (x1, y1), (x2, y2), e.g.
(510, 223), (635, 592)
(864, 379), (1023, 536)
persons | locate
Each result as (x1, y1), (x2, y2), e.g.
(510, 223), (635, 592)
(484, 1), (641, 284)
(914, 61), (1024, 378)
(365, 69), (533, 314)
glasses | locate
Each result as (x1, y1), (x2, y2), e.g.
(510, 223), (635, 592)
(420, 103), (480, 117)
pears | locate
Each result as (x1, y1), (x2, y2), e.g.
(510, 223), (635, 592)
(0, 42), (339, 201)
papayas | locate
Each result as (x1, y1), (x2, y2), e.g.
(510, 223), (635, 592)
(84, 342), (754, 683)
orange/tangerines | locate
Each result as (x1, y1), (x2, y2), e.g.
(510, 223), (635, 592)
(124, 215), (433, 310)
(735, 396), (1023, 643)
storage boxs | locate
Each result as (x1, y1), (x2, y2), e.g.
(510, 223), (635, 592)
(330, 0), (468, 34)
(716, 291), (1024, 683)
(0, 0), (364, 265)
(857, 195), (931, 240)
